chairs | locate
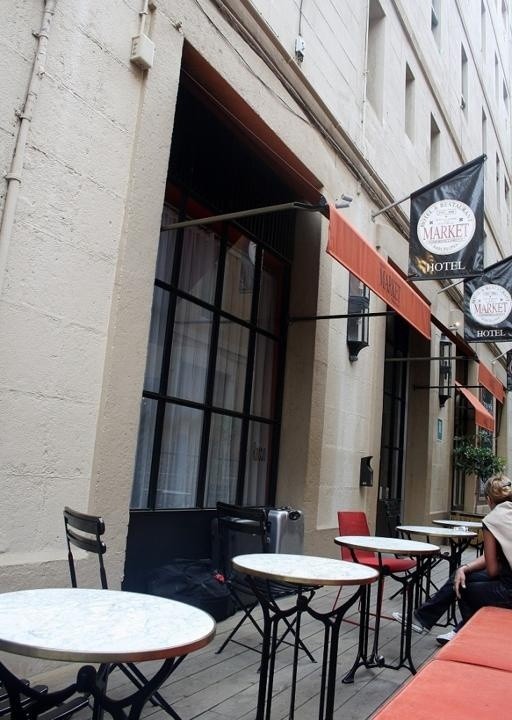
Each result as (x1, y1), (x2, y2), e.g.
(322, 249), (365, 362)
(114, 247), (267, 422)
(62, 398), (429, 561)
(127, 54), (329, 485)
(216, 498), (443, 669)
(59, 508), (181, 719)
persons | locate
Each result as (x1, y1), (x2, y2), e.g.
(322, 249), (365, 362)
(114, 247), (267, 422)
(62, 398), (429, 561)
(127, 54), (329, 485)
(434, 475), (511, 644)
(391, 554), (486, 634)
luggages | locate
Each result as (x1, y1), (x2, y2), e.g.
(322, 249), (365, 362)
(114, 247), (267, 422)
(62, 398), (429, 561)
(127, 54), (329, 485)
(209, 504), (304, 571)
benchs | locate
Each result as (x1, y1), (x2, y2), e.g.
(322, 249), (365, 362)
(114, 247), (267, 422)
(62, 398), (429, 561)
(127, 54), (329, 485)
(370, 606), (511, 720)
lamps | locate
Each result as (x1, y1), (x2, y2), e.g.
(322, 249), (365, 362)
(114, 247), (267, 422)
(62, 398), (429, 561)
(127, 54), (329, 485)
(440, 332), (454, 408)
(347, 271), (371, 363)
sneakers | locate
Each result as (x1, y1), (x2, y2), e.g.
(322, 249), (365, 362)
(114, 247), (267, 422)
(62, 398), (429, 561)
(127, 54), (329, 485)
(436, 631), (456, 645)
(392, 611), (424, 634)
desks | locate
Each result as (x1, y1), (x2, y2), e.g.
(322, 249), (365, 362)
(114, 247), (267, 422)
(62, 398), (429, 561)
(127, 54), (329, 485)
(2, 586), (216, 718)
(229, 518), (484, 720)
